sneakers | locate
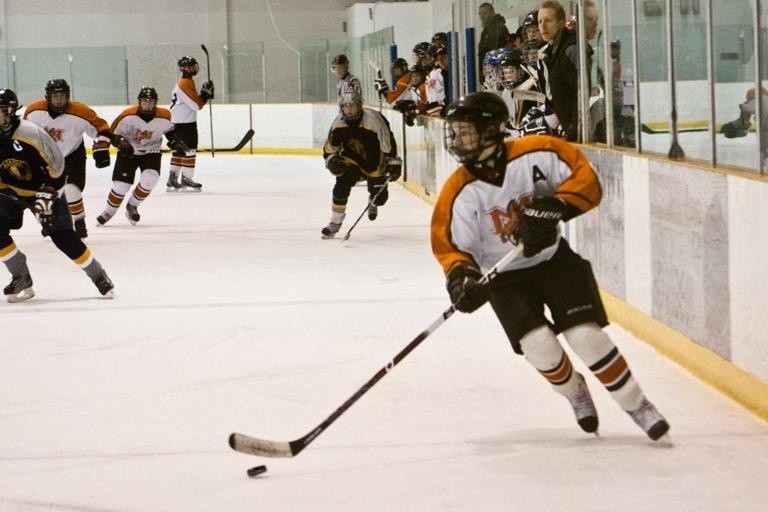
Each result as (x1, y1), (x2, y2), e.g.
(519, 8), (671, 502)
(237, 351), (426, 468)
(126, 204), (140, 222)
(96, 269), (113, 296)
(368, 196), (378, 222)
(4, 273), (34, 295)
(321, 221), (342, 236)
(567, 373), (598, 433)
(75, 217), (88, 238)
(626, 397), (669, 440)
(97, 212), (112, 225)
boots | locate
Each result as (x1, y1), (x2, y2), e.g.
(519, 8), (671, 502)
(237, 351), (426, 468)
(166, 170), (183, 189)
(181, 172), (202, 188)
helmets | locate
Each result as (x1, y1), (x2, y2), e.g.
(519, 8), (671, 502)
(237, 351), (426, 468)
(45, 79), (71, 115)
(485, 11), (549, 89)
(0, 89), (22, 127)
(136, 87), (159, 117)
(178, 56), (199, 76)
(440, 90), (508, 164)
(390, 32), (448, 86)
(329, 54), (350, 79)
(338, 90), (364, 122)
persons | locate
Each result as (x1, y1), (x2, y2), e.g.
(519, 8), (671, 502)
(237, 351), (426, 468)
(331, 54), (362, 103)
(0, 87), (114, 296)
(24, 79), (114, 238)
(321, 89), (402, 238)
(110, 87), (178, 226)
(430, 90), (670, 441)
(720, 87), (768, 158)
(373, 0), (635, 149)
(166, 56), (214, 189)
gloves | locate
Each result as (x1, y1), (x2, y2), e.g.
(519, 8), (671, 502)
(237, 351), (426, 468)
(402, 109), (417, 127)
(393, 100), (416, 112)
(326, 153), (349, 176)
(518, 115), (553, 137)
(167, 140), (190, 157)
(113, 135), (134, 159)
(518, 106), (545, 129)
(498, 195), (569, 256)
(200, 81), (214, 99)
(443, 258), (490, 314)
(385, 157), (403, 182)
(374, 79), (389, 93)
(34, 186), (56, 227)
(92, 144), (110, 168)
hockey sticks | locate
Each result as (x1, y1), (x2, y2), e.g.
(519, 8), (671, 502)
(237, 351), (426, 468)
(161, 129), (255, 153)
(639, 123), (756, 135)
(228, 242), (525, 458)
(510, 89), (574, 140)
(201, 44), (214, 158)
(345, 177), (394, 239)
